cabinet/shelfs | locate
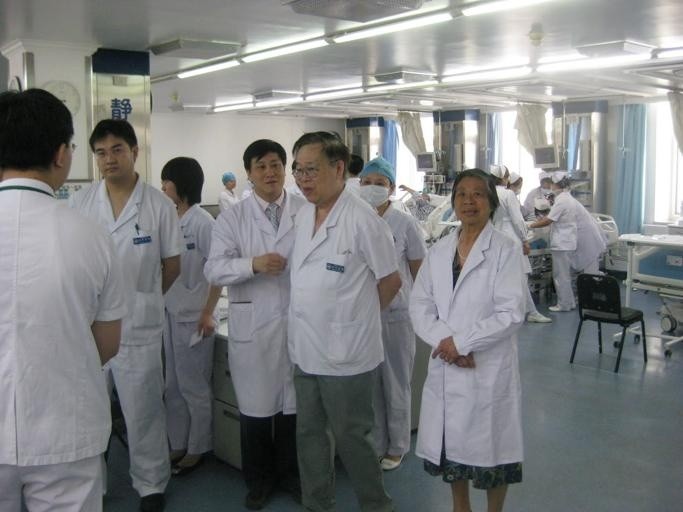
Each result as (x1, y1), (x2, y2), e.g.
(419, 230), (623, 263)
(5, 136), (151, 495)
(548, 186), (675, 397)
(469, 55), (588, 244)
(213, 320), (242, 474)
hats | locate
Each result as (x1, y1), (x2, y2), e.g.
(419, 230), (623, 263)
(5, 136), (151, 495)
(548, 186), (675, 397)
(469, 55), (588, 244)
(491, 165), (506, 179)
(539, 172), (552, 181)
(551, 171), (564, 184)
(222, 172), (235, 184)
(360, 157), (395, 187)
(534, 196), (551, 211)
(509, 172), (519, 184)
(564, 172), (570, 180)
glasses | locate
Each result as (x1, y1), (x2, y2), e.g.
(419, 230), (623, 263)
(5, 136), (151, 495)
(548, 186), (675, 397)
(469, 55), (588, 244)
(72, 142), (76, 152)
(293, 167), (319, 178)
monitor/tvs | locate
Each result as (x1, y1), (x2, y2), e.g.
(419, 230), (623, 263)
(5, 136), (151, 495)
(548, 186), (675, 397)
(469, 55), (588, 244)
(533, 145), (559, 168)
(416, 152), (436, 172)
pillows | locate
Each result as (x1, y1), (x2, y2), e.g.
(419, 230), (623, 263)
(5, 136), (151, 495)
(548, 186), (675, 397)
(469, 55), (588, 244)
(428, 194), (452, 208)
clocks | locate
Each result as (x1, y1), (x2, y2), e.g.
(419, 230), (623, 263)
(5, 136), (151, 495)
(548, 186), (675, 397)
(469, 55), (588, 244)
(43, 80), (81, 119)
(8, 77), (21, 93)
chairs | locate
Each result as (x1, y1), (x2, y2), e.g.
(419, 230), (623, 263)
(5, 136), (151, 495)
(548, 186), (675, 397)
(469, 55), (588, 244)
(569, 273), (648, 373)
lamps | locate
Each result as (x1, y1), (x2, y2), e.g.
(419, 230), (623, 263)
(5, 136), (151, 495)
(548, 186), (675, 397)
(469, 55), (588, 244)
(366, 79), (439, 94)
(305, 88), (364, 102)
(461, 0), (547, 17)
(657, 47), (683, 60)
(442, 65), (531, 85)
(241, 38), (330, 64)
(332, 10), (453, 45)
(214, 102), (254, 114)
(255, 96), (303, 108)
(536, 50), (652, 73)
(176, 59), (241, 80)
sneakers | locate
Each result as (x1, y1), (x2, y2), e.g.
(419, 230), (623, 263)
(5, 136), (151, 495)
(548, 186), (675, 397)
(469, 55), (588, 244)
(245, 489), (272, 510)
(549, 306), (559, 312)
(381, 453), (404, 470)
(571, 304), (576, 309)
(171, 451), (213, 476)
(290, 485), (302, 504)
(527, 315), (552, 323)
(170, 449), (187, 464)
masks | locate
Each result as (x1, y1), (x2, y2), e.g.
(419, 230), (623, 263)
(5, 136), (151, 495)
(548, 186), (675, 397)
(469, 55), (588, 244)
(359, 185), (390, 208)
(515, 189), (521, 195)
(541, 188), (552, 195)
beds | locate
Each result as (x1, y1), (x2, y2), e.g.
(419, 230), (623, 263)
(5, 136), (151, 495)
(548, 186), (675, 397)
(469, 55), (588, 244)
(612, 231), (683, 356)
(391, 190), (458, 246)
(435, 212), (619, 305)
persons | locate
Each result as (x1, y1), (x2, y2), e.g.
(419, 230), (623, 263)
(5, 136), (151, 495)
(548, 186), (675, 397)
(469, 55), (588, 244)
(530, 171), (577, 311)
(64, 120), (183, 512)
(355, 158), (428, 471)
(489, 165), (551, 322)
(505, 172), (526, 231)
(0, 87), (124, 512)
(204, 138), (306, 512)
(156, 156), (221, 469)
(218, 172), (239, 215)
(523, 170), (557, 231)
(408, 169), (527, 512)
(288, 131), (402, 512)
(345, 155), (364, 193)
(534, 193), (606, 301)
(399, 185), (422, 200)
(417, 192), (456, 222)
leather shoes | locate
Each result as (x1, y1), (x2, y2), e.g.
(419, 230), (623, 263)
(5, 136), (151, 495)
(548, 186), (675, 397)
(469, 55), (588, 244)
(139, 493), (166, 512)
